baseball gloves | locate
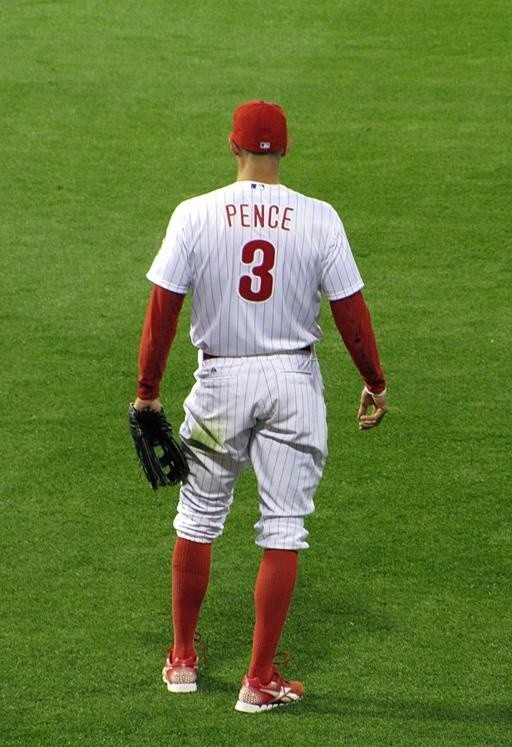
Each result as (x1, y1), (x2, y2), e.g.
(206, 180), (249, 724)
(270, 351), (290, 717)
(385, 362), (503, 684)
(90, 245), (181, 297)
(129, 400), (191, 490)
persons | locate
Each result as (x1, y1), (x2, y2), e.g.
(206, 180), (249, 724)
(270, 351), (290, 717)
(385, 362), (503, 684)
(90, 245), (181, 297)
(134, 100), (388, 714)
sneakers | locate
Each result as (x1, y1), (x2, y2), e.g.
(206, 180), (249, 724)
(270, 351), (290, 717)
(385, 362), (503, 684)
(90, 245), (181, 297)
(161, 644), (199, 694)
(234, 669), (305, 714)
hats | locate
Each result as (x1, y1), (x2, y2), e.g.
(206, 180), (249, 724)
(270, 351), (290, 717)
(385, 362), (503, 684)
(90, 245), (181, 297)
(230, 99), (288, 156)
